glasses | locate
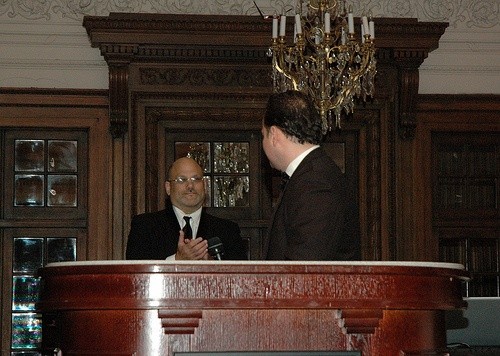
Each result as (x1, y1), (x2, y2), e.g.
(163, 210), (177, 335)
(168, 175), (204, 184)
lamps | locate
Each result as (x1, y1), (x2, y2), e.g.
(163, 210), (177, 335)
(265, 0), (379, 136)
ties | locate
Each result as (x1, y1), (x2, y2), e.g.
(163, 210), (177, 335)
(182, 216), (192, 242)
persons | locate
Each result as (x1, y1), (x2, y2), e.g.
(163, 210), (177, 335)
(126, 157), (240, 261)
(261, 90), (360, 261)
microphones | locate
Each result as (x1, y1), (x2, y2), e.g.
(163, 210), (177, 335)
(207, 236), (225, 260)
